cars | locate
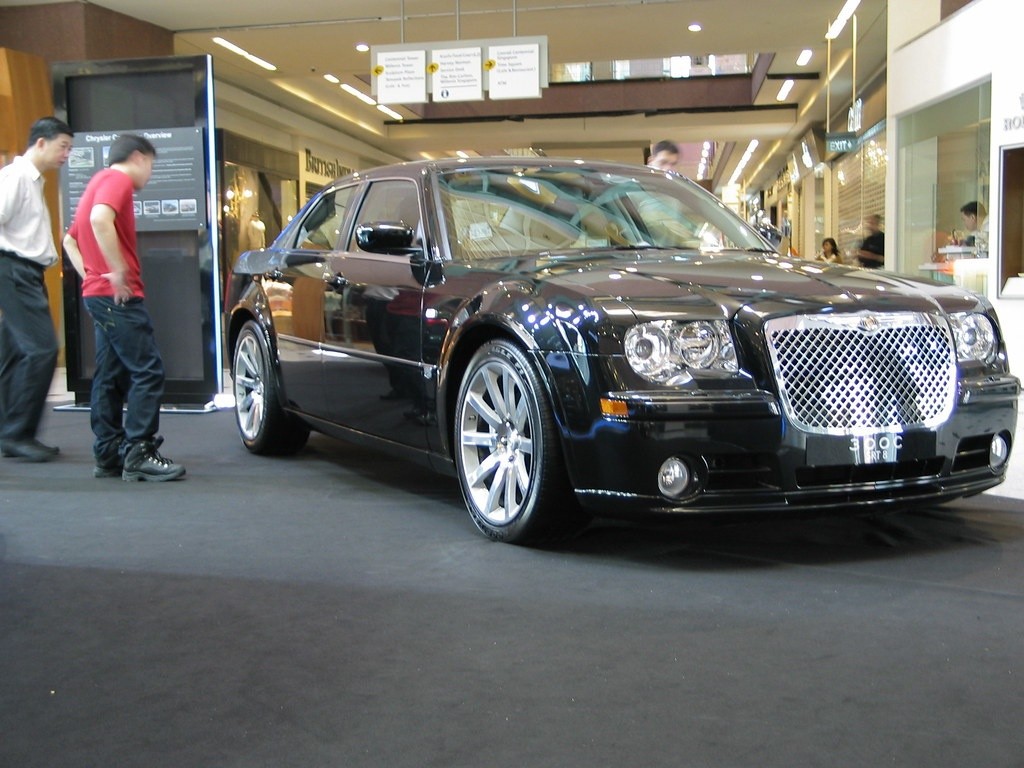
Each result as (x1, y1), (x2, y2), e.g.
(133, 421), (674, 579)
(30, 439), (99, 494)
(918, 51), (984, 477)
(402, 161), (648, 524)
(225, 152), (1024, 546)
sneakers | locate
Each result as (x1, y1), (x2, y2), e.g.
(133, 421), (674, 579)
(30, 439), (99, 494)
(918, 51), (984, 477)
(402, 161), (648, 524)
(122, 436), (186, 482)
(94, 436), (124, 478)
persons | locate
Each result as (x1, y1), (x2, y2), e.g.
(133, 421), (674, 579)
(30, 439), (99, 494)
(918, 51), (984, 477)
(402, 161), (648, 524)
(960, 200), (989, 259)
(61, 134), (187, 484)
(815, 237), (841, 264)
(854, 213), (885, 269)
(224, 208), (239, 276)
(248, 212), (266, 250)
(0, 117), (76, 464)
(646, 139), (681, 171)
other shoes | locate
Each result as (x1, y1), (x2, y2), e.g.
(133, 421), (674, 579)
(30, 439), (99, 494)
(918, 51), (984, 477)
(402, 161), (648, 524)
(0, 437), (60, 462)
